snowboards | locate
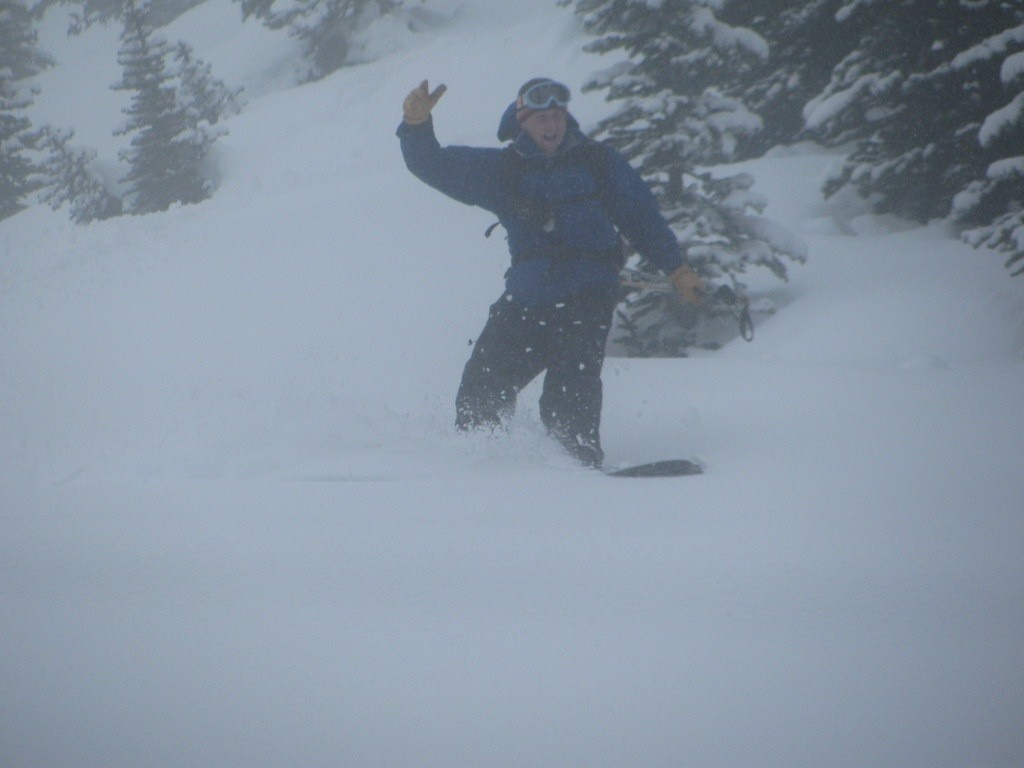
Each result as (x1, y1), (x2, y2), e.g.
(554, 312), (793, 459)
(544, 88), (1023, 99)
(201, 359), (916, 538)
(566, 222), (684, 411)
(586, 459), (704, 477)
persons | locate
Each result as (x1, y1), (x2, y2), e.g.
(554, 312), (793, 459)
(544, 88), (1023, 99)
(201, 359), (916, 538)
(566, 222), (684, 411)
(396, 78), (705, 466)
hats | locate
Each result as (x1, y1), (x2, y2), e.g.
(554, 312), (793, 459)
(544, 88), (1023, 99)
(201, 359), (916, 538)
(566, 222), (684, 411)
(516, 78), (559, 125)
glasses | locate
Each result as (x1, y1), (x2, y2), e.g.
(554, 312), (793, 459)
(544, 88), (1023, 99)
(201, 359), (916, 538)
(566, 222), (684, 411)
(524, 81), (572, 108)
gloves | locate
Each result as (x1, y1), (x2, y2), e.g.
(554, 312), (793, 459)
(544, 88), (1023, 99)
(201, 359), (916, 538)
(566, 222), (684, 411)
(670, 268), (708, 310)
(402, 79), (447, 125)
